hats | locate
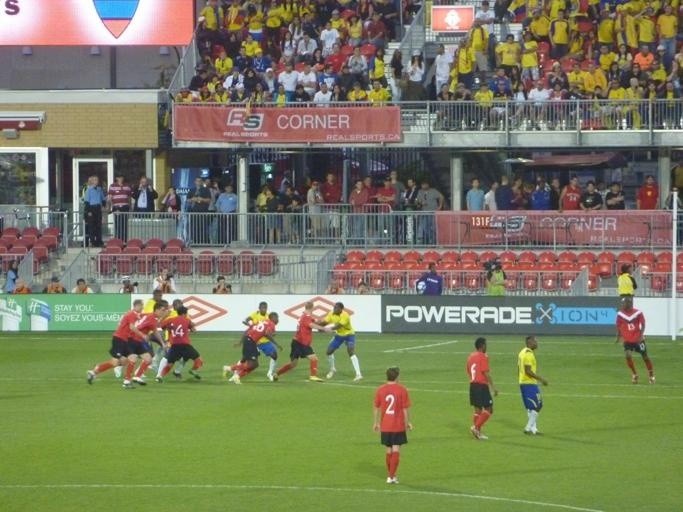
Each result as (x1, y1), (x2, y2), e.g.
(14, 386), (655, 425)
(621, 263), (631, 272)
(51, 277), (60, 283)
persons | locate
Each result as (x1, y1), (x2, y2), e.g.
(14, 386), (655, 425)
(216, 184), (238, 245)
(416, 179), (444, 245)
(176, 2), (394, 105)
(205, 179), (221, 225)
(466, 337), (498, 441)
(559, 175), (582, 213)
(467, 178), (485, 210)
(364, 177), (381, 212)
(223, 313), (283, 384)
(616, 294), (656, 384)
(402, 176), (419, 245)
(390, 171), (406, 244)
(153, 268), (177, 293)
(377, 177), (396, 246)
(121, 302), (169, 386)
(7, 260), (18, 293)
(108, 173), (134, 243)
(81, 175), (107, 248)
(213, 276), (234, 294)
(637, 175), (659, 210)
(134, 176), (158, 219)
(157, 299), (192, 378)
(433, 2), (681, 130)
(350, 180), (369, 245)
(246, 302), (278, 382)
(416, 262), (442, 294)
(43, 277), (68, 293)
(12, 279), (32, 293)
(187, 177), (212, 244)
(257, 172), (342, 244)
(606, 181), (625, 209)
(407, 49), (426, 102)
(374, 367), (415, 484)
(325, 280), (342, 292)
(157, 307), (204, 384)
(486, 258), (507, 296)
(86, 299), (150, 389)
(316, 303), (364, 382)
(72, 279), (94, 293)
(518, 335), (549, 435)
(353, 280), (370, 294)
(580, 180), (604, 211)
(276, 301), (327, 381)
(162, 186), (181, 218)
(143, 290), (166, 373)
(597, 181), (611, 210)
(119, 276), (138, 293)
(484, 175), (563, 210)
(617, 262), (638, 296)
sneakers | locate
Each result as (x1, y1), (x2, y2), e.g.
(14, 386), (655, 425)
(308, 376), (324, 382)
(87, 370), (93, 385)
(631, 374), (639, 384)
(132, 376), (146, 386)
(228, 374), (237, 382)
(353, 375), (363, 383)
(188, 369), (202, 380)
(326, 368), (337, 380)
(266, 372), (274, 382)
(524, 429), (532, 434)
(122, 383), (135, 389)
(386, 477), (392, 485)
(535, 431), (543, 435)
(649, 375), (655, 384)
(272, 371), (279, 382)
(113, 366), (122, 380)
(134, 370), (146, 378)
(154, 377), (163, 384)
(470, 426), (480, 439)
(172, 370), (183, 379)
(479, 433), (489, 440)
(232, 374), (242, 386)
(392, 477), (399, 484)
(222, 365), (229, 379)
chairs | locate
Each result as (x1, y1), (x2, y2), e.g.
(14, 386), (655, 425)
(327, 251), (682, 292)
(0, 226), (58, 278)
(95, 238), (279, 277)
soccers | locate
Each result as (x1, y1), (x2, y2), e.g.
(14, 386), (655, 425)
(417, 281), (426, 291)
